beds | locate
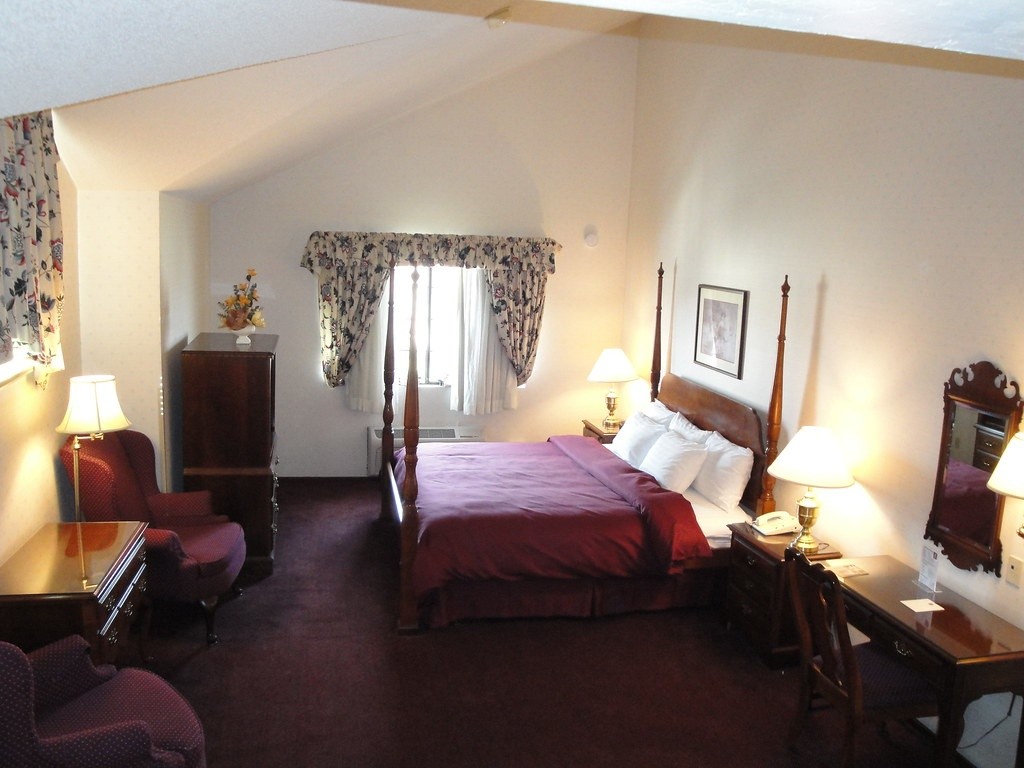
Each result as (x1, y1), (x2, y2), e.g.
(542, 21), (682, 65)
(379, 252), (793, 640)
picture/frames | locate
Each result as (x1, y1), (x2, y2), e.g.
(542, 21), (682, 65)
(688, 283), (749, 381)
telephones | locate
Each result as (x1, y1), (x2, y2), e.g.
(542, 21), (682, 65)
(752, 510), (802, 536)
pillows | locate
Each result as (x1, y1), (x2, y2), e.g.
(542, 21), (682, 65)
(682, 429), (757, 514)
(671, 410), (715, 445)
(643, 398), (677, 427)
(604, 412), (664, 468)
(638, 432), (707, 493)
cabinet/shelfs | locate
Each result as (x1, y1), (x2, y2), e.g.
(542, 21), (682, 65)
(178, 330), (281, 580)
(971, 423), (1004, 473)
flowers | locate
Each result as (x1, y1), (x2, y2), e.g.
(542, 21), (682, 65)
(218, 268), (265, 332)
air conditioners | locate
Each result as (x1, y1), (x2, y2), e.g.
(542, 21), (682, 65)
(367, 426), (484, 478)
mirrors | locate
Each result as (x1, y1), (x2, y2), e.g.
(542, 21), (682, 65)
(923, 361), (1023, 577)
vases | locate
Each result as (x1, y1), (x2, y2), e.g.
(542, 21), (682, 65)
(236, 323), (255, 343)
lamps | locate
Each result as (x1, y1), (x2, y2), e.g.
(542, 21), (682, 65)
(986, 417), (1023, 538)
(40, 372), (131, 584)
(767, 428), (849, 556)
(585, 347), (637, 428)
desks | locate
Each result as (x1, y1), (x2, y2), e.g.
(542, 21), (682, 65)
(788, 554), (1024, 768)
(1, 518), (154, 661)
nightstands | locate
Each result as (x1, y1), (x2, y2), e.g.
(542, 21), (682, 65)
(715, 522), (839, 669)
(581, 418), (631, 445)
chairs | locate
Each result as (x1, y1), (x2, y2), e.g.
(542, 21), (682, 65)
(782, 547), (947, 767)
(61, 431), (245, 645)
(0, 635), (211, 768)
